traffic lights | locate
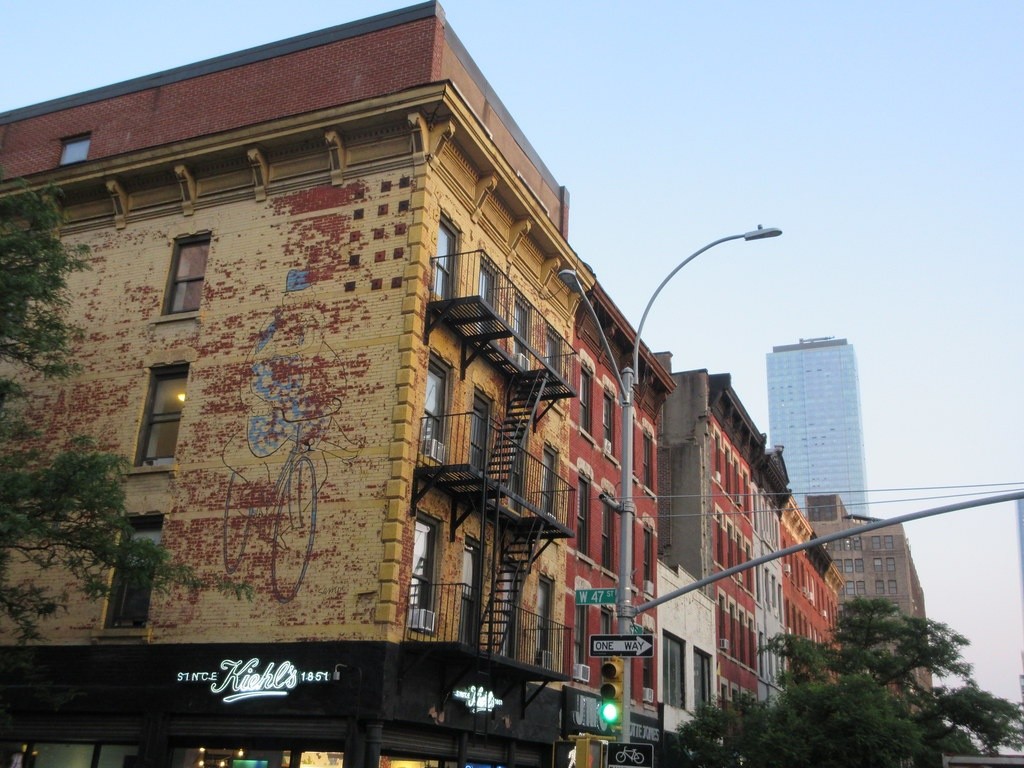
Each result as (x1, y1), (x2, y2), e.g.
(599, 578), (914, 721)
(600, 658), (623, 724)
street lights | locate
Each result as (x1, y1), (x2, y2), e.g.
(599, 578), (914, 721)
(556, 224), (783, 744)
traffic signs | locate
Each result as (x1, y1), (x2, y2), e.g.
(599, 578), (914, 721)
(589, 633), (655, 658)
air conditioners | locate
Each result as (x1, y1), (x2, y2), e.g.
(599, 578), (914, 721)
(784, 564), (790, 573)
(426, 439), (445, 464)
(642, 688), (654, 701)
(573, 664), (590, 682)
(736, 495), (743, 506)
(514, 353), (531, 371)
(411, 610), (435, 632)
(535, 650), (553, 670)
(720, 639), (728, 649)
(645, 579), (654, 594)
